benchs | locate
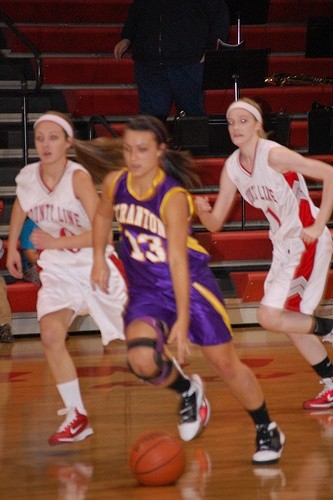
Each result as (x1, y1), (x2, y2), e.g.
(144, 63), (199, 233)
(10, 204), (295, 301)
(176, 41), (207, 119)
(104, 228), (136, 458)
(0, 0), (333, 336)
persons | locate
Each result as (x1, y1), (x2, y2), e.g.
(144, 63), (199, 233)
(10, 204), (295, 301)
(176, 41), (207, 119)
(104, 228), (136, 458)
(6, 112), (132, 446)
(90, 117), (285, 462)
(195, 98), (333, 411)
(114, 0), (213, 117)
(0, 240), (14, 344)
(19, 218), (41, 285)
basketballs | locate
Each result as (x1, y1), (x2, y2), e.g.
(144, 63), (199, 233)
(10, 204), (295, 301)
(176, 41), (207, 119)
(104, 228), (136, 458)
(128, 430), (186, 487)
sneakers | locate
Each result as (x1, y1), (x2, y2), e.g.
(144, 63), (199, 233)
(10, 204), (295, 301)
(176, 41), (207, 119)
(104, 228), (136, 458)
(177, 380), (201, 442)
(252, 421), (286, 463)
(304, 381), (333, 409)
(200, 397), (210, 424)
(48, 407), (93, 446)
